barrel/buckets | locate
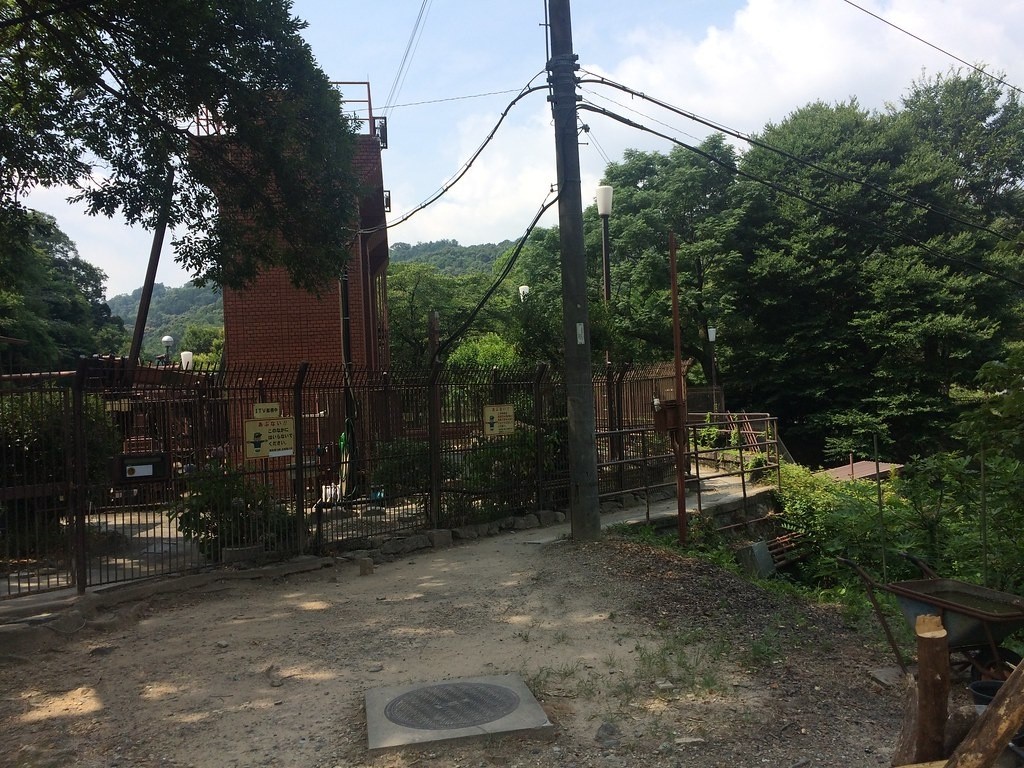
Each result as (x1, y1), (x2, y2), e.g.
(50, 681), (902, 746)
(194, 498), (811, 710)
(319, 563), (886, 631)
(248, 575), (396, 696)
(968, 679), (1007, 705)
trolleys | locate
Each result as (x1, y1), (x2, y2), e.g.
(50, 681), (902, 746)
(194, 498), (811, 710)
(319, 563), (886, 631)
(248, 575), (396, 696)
(836, 547), (1024, 692)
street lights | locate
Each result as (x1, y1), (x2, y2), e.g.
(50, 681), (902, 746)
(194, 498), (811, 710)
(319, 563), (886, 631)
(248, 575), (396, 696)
(594, 183), (615, 465)
